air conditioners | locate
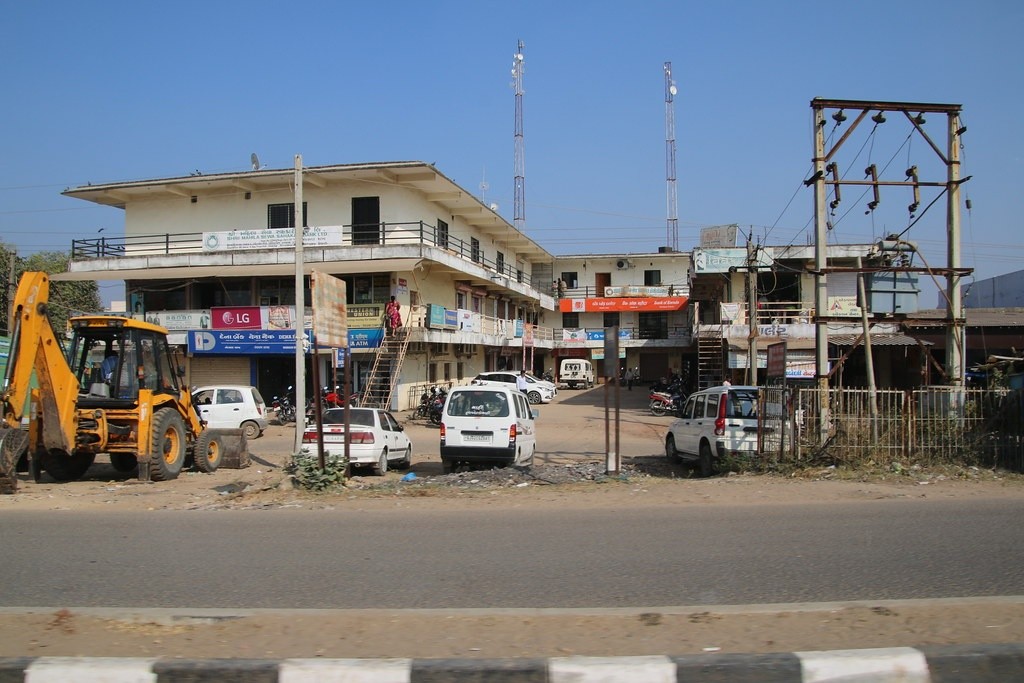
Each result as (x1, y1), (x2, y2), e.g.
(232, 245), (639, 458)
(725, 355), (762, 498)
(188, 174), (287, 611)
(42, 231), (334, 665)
(409, 342), (427, 352)
(436, 343), (449, 353)
(463, 344), (478, 354)
(616, 259), (629, 270)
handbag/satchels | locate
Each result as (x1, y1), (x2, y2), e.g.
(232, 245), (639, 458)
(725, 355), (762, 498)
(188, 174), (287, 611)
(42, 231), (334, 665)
(387, 302), (394, 318)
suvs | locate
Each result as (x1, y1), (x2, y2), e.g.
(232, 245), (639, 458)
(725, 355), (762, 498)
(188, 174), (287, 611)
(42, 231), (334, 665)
(665, 385), (791, 477)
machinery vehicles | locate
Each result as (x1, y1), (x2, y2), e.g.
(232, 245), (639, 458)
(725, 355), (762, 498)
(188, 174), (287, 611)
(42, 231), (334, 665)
(0, 271), (252, 494)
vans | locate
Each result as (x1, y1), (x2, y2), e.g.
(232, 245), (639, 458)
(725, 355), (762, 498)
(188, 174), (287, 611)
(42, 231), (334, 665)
(440, 386), (535, 472)
(560, 359), (595, 390)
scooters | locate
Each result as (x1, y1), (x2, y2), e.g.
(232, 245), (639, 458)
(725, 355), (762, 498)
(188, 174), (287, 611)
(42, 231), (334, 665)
(649, 369), (688, 417)
(413, 384), (450, 425)
(272, 386), (358, 426)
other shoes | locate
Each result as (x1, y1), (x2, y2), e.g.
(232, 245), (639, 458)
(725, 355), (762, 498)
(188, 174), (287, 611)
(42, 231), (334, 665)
(392, 335), (396, 337)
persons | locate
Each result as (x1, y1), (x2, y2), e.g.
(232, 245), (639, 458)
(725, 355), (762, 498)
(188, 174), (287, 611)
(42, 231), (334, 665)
(759, 293), (769, 324)
(200, 311), (209, 328)
(546, 368), (553, 382)
(696, 253), (705, 270)
(101, 351), (119, 382)
(386, 296), (402, 337)
(147, 312), (160, 325)
(516, 369), (528, 395)
(552, 278), (567, 298)
(626, 368), (633, 391)
(132, 301), (144, 322)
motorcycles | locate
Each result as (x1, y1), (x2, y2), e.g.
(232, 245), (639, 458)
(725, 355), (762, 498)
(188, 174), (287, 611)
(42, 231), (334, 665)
(542, 367), (554, 382)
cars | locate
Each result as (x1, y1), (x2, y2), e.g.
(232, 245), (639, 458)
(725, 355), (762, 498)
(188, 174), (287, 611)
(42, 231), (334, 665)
(471, 370), (558, 405)
(192, 385), (267, 440)
(301, 408), (412, 476)
